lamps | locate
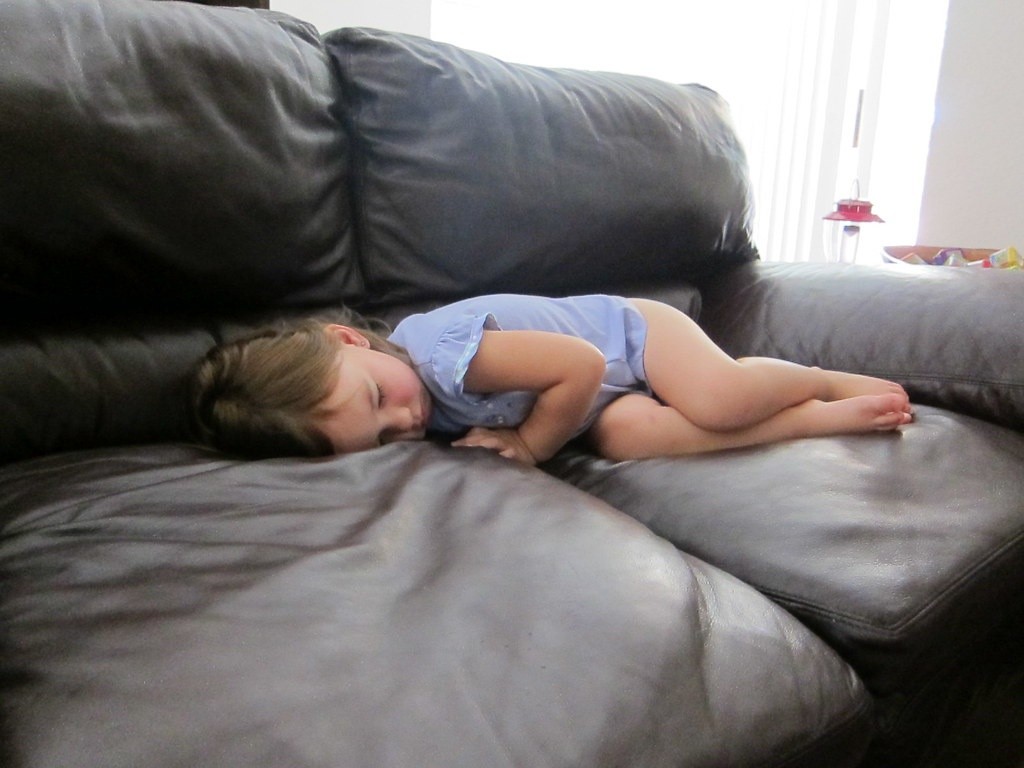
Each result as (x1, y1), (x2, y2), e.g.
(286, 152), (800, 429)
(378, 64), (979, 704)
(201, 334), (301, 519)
(820, 147), (883, 257)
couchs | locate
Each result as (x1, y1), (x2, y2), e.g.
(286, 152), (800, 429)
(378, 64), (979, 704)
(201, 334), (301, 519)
(0, 0), (1024, 768)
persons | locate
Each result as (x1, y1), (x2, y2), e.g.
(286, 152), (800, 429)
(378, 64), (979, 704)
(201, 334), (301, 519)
(178, 293), (914, 467)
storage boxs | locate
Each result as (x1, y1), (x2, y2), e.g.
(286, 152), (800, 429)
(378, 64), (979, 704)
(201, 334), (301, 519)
(879, 245), (1024, 267)
